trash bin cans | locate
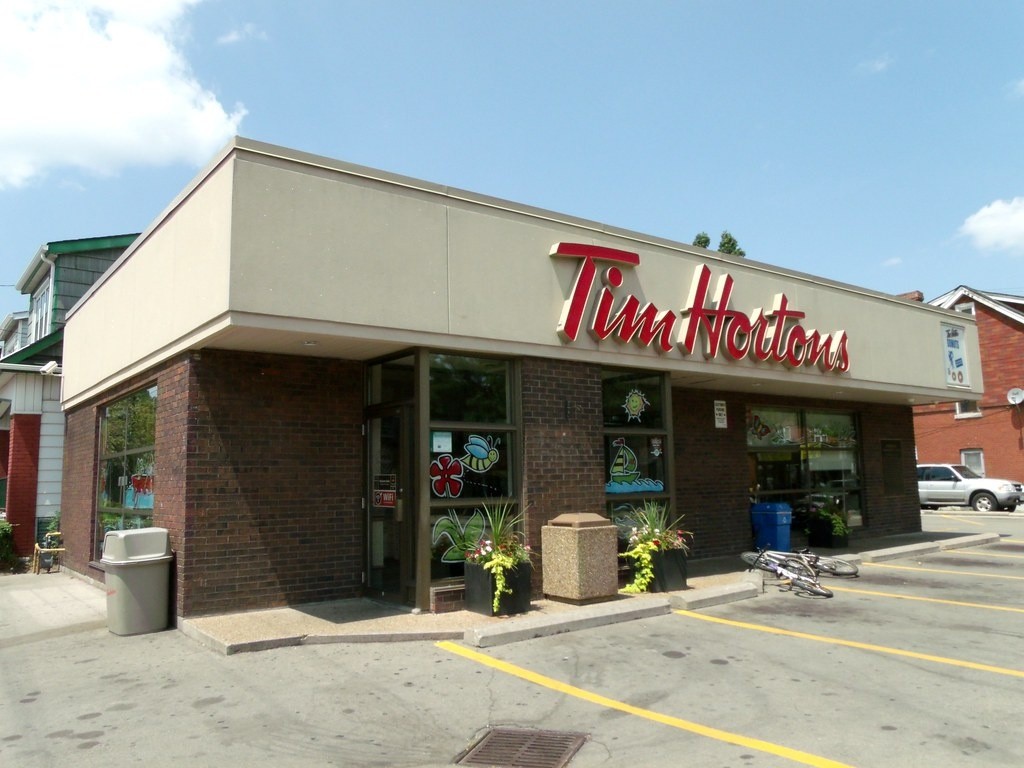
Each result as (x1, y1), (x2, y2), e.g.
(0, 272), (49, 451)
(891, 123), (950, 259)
(99, 526), (174, 637)
(750, 502), (793, 552)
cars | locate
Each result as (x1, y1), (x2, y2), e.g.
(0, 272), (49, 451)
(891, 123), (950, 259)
(806, 480), (859, 512)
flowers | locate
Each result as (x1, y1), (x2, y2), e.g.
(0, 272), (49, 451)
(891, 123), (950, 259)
(798, 502), (854, 538)
(458, 491), (542, 612)
(616, 496), (694, 595)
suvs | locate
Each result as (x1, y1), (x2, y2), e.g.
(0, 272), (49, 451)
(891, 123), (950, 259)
(915, 464), (1024, 513)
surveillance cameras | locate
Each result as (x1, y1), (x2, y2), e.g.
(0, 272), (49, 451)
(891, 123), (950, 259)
(41, 361), (58, 375)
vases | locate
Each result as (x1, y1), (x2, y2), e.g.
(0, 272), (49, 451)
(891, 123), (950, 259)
(630, 549), (688, 593)
(808, 518), (849, 549)
(464, 561), (532, 617)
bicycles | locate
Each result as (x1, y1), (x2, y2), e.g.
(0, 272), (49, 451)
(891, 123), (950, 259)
(740, 542), (860, 597)
